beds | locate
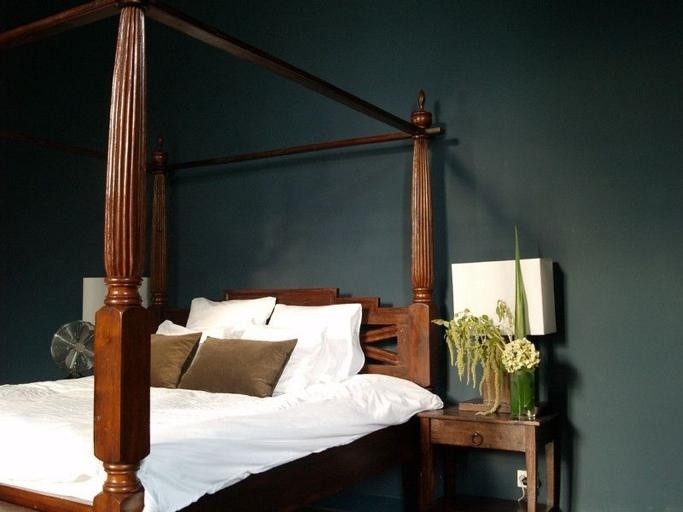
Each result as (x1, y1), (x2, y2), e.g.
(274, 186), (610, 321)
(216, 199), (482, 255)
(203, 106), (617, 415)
(0, 0), (444, 512)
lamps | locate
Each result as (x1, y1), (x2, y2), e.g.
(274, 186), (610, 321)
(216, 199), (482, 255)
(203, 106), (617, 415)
(451, 258), (556, 414)
(81, 276), (151, 328)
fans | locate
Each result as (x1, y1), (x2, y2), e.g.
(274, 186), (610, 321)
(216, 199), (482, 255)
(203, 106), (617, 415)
(50, 320), (95, 378)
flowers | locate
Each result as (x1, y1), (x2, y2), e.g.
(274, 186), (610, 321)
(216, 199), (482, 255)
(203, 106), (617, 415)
(433, 226), (542, 417)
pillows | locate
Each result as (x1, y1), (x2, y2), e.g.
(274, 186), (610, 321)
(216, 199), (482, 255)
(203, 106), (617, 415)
(147, 297), (365, 397)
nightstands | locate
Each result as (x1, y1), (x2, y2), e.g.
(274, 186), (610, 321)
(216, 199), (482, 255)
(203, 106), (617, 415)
(417, 402), (562, 512)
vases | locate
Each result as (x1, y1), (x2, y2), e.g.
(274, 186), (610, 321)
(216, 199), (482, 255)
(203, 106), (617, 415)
(509, 372), (534, 420)
